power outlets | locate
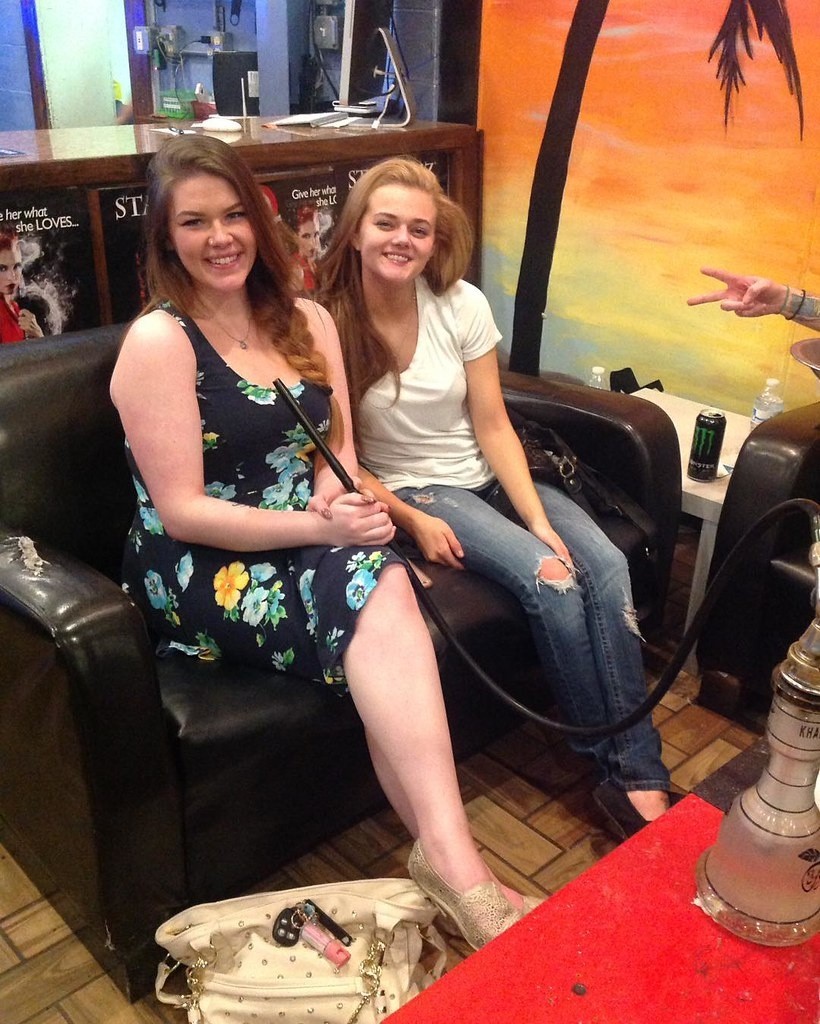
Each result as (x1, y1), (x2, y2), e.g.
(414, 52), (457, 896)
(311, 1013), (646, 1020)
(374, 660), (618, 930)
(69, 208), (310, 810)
(207, 32), (225, 51)
(158, 25), (179, 53)
(133, 26), (152, 55)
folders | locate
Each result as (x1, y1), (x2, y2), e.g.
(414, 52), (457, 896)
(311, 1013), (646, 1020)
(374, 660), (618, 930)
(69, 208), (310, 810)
(268, 111), (349, 129)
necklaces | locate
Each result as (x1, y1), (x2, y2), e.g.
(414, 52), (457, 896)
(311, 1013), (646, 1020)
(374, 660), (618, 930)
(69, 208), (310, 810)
(214, 316), (251, 350)
(394, 296), (416, 367)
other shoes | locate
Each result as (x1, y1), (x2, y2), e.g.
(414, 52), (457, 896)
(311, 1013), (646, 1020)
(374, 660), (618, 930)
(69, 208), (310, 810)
(407, 838), (524, 951)
(592, 778), (681, 842)
(440, 894), (545, 938)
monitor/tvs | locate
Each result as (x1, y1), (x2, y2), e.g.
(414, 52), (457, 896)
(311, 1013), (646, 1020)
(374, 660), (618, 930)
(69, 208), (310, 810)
(339, 0), (415, 127)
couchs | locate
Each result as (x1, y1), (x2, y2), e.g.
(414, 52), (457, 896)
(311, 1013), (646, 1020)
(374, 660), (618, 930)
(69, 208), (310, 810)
(697, 401), (820, 733)
(0, 322), (685, 1002)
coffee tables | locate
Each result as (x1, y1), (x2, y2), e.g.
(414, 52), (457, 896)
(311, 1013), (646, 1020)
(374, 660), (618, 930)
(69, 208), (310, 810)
(380, 736), (820, 1024)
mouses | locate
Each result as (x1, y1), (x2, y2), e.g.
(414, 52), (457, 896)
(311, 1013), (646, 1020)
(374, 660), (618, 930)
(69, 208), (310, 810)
(202, 117), (242, 132)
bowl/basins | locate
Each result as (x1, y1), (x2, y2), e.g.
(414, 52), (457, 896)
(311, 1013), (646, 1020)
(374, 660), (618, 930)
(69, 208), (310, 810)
(790, 338), (820, 380)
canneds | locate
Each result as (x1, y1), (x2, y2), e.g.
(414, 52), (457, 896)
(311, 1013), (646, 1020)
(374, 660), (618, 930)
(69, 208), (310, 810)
(687, 409), (726, 482)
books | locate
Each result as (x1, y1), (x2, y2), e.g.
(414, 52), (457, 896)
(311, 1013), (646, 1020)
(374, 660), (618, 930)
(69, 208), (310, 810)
(0, 148), (449, 335)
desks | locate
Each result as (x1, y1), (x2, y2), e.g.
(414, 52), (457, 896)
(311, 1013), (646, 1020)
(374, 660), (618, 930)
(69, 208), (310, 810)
(0, 116), (478, 345)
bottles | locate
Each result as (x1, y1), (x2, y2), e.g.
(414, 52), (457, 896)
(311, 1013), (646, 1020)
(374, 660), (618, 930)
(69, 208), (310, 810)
(585, 366), (608, 390)
(749, 377), (784, 433)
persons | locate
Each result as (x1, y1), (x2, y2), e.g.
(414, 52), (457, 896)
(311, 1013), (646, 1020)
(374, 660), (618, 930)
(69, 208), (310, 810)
(108, 136), (548, 953)
(0, 229), (45, 343)
(286, 203), (322, 292)
(687, 264), (820, 332)
(314, 153), (684, 839)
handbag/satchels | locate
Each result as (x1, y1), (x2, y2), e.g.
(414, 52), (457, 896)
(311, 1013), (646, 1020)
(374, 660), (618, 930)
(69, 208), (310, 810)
(155, 879), (449, 1024)
(508, 411), (627, 524)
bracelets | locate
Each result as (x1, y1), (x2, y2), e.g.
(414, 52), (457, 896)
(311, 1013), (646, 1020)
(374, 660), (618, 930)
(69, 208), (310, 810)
(785, 289), (806, 320)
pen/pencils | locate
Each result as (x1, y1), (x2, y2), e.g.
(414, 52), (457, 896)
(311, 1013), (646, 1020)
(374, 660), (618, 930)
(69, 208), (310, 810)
(170, 127), (177, 132)
(179, 128), (184, 134)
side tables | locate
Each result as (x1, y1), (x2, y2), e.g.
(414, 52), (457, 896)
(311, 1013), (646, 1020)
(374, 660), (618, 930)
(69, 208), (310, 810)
(629, 388), (752, 675)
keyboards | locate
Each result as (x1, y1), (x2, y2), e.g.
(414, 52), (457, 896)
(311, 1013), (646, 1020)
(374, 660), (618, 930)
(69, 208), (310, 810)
(276, 111), (347, 128)
